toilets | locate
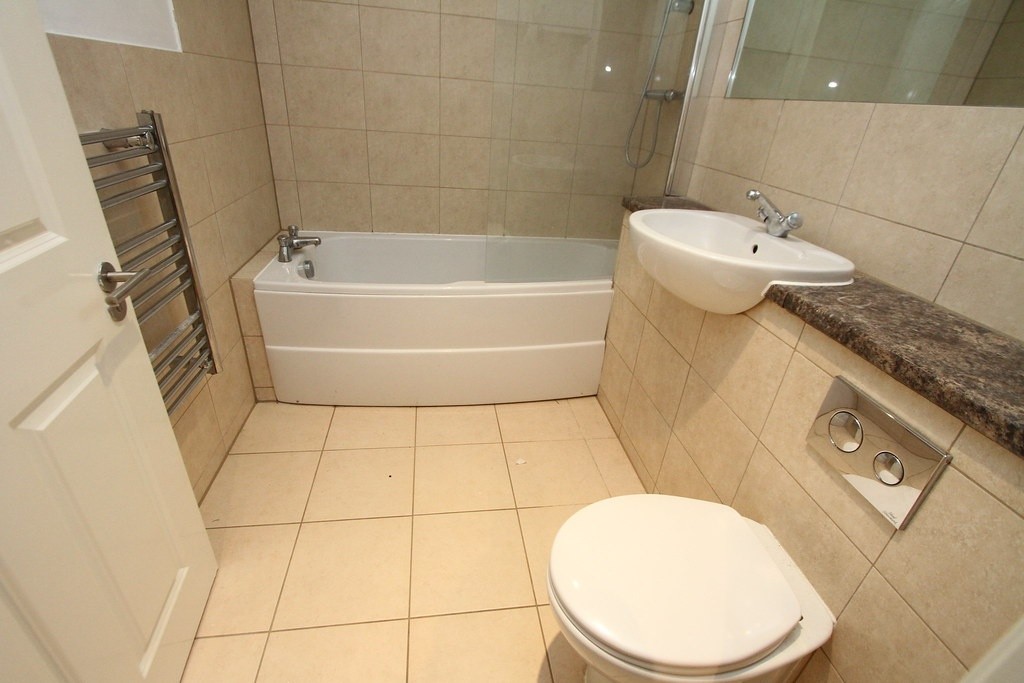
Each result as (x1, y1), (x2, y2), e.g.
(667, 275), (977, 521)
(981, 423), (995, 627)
(547, 493), (838, 682)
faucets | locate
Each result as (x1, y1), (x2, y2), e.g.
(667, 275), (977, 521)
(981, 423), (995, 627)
(288, 234), (323, 251)
(746, 188), (803, 237)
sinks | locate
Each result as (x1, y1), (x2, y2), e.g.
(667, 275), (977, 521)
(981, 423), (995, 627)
(628, 207), (857, 314)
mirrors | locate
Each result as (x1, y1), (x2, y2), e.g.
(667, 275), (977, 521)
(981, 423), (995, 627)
(723, 0), (1024, 108)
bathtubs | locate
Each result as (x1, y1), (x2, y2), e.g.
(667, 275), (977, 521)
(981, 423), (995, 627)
(253, 231), (621, 408)
